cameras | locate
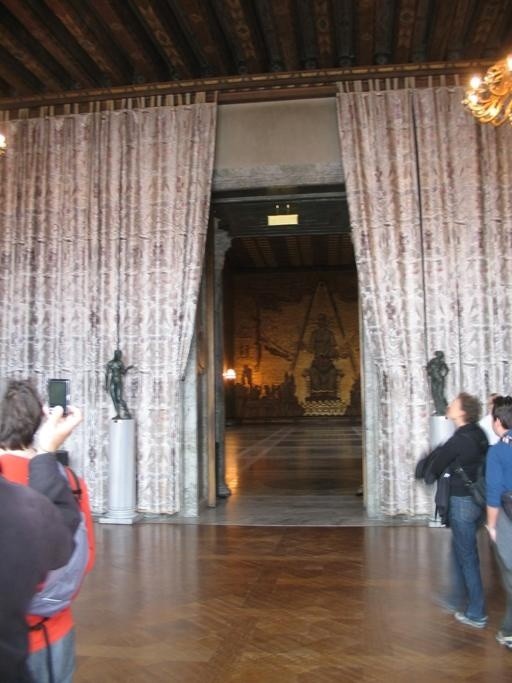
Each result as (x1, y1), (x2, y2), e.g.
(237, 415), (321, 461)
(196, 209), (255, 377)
(47, 379), (71, 415)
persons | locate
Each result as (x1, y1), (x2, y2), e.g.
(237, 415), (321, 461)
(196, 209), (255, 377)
(104, 350), (133, 419)
(477, 392), (501, 446)
(425, 392), (490, 630)
(0, 402), (84, 683)
(0, 380), (96, 683)
(306, 313), (338, 396)
(485, 395), (512, 649)
(426, 351), (449, 416)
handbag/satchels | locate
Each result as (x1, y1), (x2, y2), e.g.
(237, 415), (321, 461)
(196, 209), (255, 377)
(501, 491), (512, 522)
(468, 478), (486, 508)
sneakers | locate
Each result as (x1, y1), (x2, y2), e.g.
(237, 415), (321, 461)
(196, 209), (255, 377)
(495, 631), (512, 649)
(455, 612), (488, 629)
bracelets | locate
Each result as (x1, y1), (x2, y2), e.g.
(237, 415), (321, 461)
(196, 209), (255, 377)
(485, 526), (495, 532)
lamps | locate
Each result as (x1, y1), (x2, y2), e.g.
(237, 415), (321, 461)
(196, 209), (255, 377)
(459, 54), (512, 129)
